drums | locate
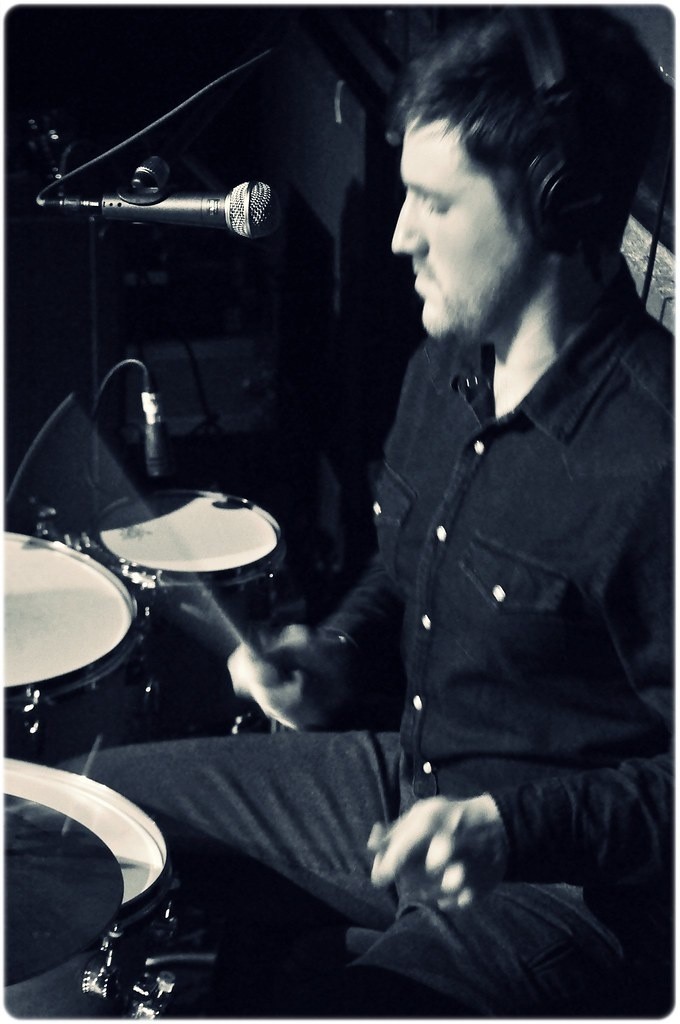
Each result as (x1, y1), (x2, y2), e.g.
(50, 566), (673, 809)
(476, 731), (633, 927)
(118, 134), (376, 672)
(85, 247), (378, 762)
(5, 530), (148, 769)
(93, 487), (289, 640)
(5, 756), (176, 1019)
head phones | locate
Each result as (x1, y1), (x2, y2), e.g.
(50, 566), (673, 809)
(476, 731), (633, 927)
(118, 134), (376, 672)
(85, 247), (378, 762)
(506, 4), (615, 250)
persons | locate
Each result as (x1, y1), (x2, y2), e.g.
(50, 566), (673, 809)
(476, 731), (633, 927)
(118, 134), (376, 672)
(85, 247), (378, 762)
(36, 5), (675, 1020)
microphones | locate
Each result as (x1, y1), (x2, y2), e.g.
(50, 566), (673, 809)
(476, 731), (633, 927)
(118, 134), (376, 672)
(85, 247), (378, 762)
(141, 373), (177, 478)
(44, 179), (280, 240)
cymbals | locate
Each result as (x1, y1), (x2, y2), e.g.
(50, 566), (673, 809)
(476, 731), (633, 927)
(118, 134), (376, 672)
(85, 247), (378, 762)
(7, 795), (125, 990)
